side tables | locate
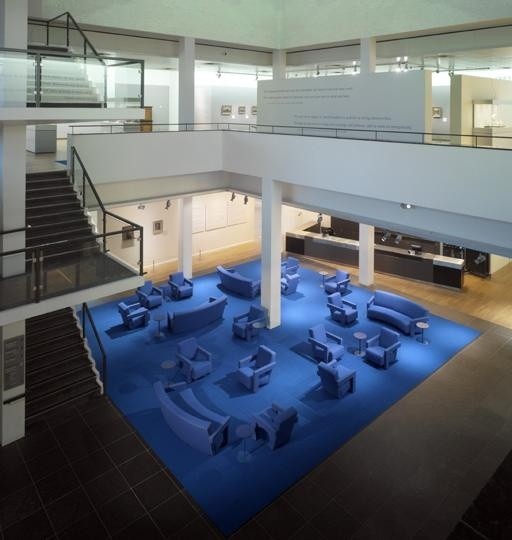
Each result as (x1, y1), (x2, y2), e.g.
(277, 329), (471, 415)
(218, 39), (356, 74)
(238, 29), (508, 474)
(236, 424), (251, 463)
(416, 321), (431, 345)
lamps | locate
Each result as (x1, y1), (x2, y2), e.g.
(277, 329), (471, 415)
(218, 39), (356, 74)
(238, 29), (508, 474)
(381, 232), (402, 244)
(138, 192), (248, 211)
(215, 56), (455, 79)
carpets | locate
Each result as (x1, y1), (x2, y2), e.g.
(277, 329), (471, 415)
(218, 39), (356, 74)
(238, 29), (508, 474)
(75, 261), (483, 540)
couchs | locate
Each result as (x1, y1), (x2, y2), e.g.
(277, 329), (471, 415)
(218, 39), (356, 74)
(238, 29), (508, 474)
(119, 301), (152, 329)
(324, 268), (352, 294)
(306, 322), (347, 363)
(175, 336), (216, 382)
(136, 280), (164, 307)
(282, 258), (301, 272)
(326, 291), (360, 325)
(255, 401), (299, 450)
(233, 304), (269, 341)
(167, 271), (195, 300)
(214, 264), (262, 300)
(367, 290), (430, 337)
(152, 380), (232, 452)
(314, 358), (359, 399)
(279, 272), (301, 296)
(237, 344), (277, 392)
(364, 326), (401, 370)
(166, 295), (229, 334)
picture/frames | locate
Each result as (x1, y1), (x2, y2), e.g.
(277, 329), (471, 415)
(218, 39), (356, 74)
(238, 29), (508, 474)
(221, 105), (257, 115)
(432, 107), (441, 118)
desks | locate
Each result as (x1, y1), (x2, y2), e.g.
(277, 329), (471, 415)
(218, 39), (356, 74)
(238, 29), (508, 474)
(318, 269), (328, 285)
(151, 314), (166, 343)
(251, 323), (267, 341)
(351, 331), (367, 359)
